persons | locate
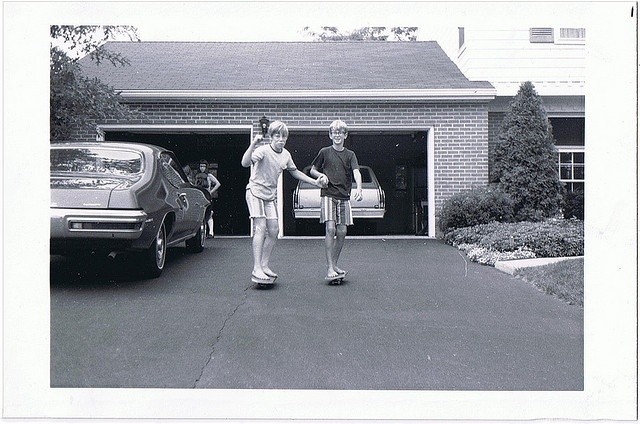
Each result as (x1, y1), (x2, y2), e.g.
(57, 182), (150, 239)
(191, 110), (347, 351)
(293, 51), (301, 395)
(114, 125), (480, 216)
(194, 159), (220, 239)
(310, 120), (363, 277)
(240, 121), (327, 280)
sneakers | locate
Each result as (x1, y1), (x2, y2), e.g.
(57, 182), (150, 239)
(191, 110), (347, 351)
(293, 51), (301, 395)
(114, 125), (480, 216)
(206, 234), (214, 239)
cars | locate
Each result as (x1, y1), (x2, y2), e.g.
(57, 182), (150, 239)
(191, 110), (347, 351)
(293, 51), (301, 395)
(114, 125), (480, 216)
(49, 141), (214, 280)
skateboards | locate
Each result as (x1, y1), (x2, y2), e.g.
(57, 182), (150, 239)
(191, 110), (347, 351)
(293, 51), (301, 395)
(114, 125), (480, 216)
(325, 269), (349, 285)
(251, 274), (277, 290)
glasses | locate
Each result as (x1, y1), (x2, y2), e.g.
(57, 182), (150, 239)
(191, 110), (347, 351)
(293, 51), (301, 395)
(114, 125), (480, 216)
(200, 165), (206, 168)
(331, 131), (345, 136)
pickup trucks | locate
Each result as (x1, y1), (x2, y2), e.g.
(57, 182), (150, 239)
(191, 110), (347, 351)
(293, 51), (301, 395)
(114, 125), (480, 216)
(292, 164), (386, 236)
